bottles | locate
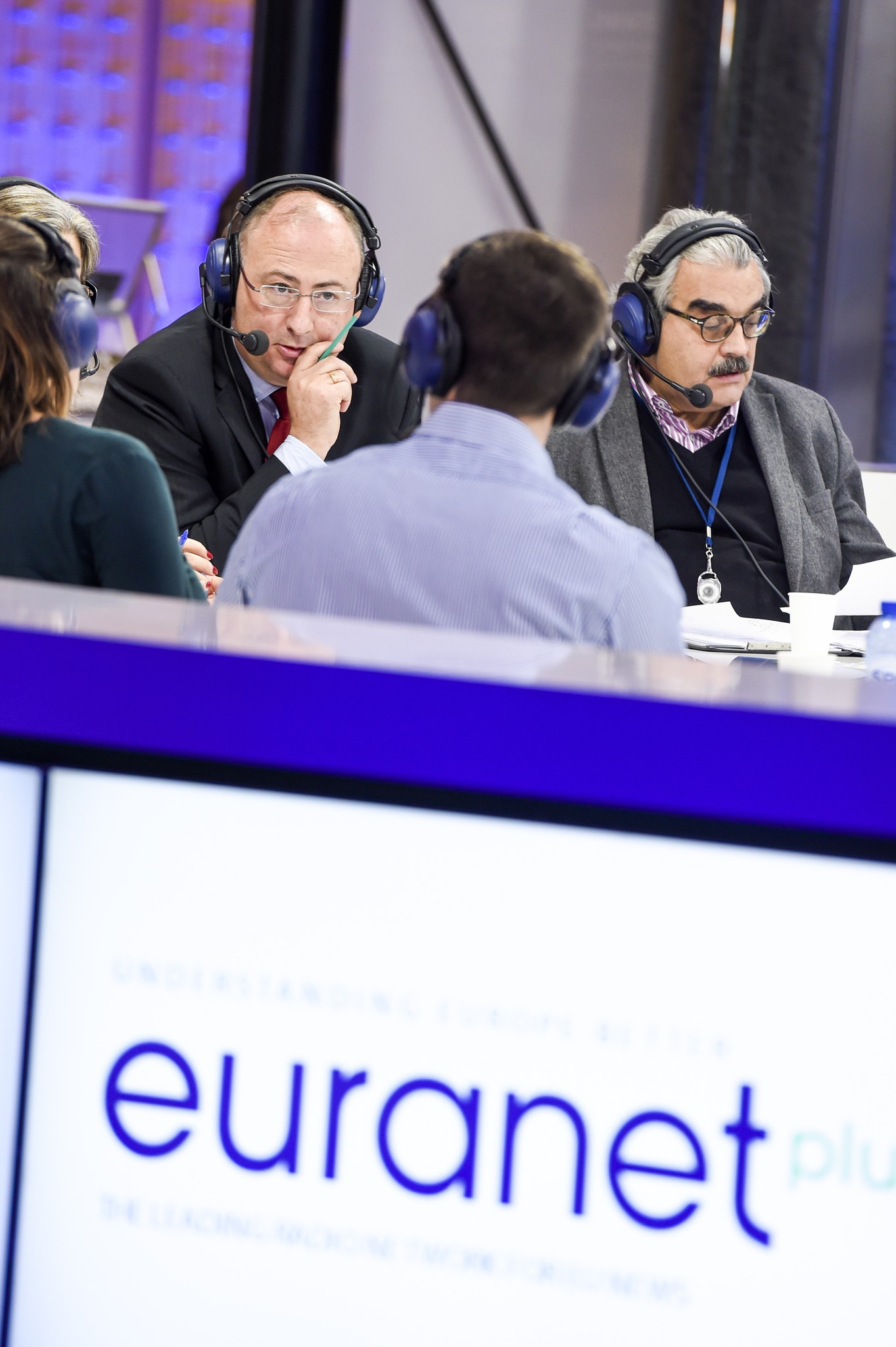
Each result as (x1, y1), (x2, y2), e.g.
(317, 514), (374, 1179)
(863, 601), (896, 683)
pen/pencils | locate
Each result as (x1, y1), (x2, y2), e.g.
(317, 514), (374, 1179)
(180, 527), (187, 548)
(317, 308), (363, 365)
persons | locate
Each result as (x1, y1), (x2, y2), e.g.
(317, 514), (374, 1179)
(543, 208), (896, 630)
(0, 177), (225, 605)
(92, 171), (426, 577)
(217, 231), (689, 658)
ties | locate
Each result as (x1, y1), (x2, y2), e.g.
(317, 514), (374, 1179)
(265, 387), (290, 461)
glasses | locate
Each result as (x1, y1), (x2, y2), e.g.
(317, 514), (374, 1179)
(240, 263), (361, 313)
(663, 305), (775, 343)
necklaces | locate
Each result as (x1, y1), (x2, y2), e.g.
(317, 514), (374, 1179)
(647, 414), (736, 603)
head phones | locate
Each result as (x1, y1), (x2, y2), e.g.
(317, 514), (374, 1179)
(401, 235), (621, 434)
(0, 214), (100, 370)
(204, 173), (385, 327)
(613, 219), (776, 357)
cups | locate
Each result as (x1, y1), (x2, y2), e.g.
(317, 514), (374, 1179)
(787, 592), (839, 659)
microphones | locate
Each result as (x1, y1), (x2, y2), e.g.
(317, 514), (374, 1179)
(610, 321), (713, 408)
(198, 264), (269, 356)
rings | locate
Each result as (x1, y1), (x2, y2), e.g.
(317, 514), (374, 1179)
(328, 372), (339, 384)
